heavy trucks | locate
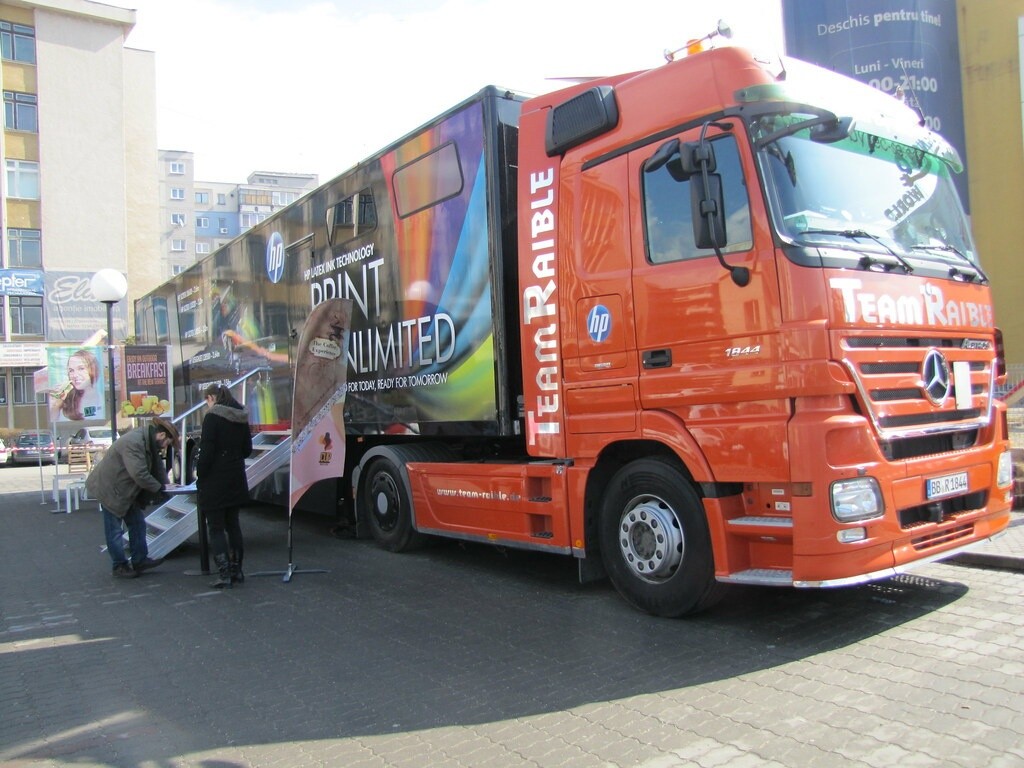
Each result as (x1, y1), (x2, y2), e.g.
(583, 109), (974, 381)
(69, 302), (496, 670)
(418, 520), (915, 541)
(121, 20), (1014, 618)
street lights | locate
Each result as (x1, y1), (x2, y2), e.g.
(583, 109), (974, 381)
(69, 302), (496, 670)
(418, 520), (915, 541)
(90, 269), (128, 443)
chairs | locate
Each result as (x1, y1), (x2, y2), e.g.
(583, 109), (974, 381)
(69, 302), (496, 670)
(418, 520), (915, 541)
(65, 455), (103, 514)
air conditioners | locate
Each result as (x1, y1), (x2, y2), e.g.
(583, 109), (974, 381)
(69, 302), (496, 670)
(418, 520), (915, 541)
(220, 228), (228, 233)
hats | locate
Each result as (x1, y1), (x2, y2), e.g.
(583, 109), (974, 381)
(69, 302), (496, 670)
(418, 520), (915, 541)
(152, 416), (180, 452)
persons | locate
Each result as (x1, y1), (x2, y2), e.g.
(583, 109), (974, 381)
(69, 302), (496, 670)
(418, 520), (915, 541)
(197, 384), (252, 588)
(50, 350), (101, 420)
(85, 416), (181, 579)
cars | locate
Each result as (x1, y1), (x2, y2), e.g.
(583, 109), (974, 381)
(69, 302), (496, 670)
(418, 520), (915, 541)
(66, 425), (121, 462)
(11, 427), (62, 466)
(0, 440), (8, 469)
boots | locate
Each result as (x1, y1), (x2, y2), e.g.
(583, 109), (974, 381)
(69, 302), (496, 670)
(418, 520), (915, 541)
(229, 545), (245, 584)
(208, 552), (232, 589)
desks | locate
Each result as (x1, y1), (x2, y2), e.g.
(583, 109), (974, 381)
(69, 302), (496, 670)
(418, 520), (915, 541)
(160, 477), (217, 578)
(53, 473), (86, 502)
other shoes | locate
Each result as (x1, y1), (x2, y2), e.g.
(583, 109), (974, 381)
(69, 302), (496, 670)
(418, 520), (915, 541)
(132, 557), (164, 572)
(113, 562), (137, 578)
